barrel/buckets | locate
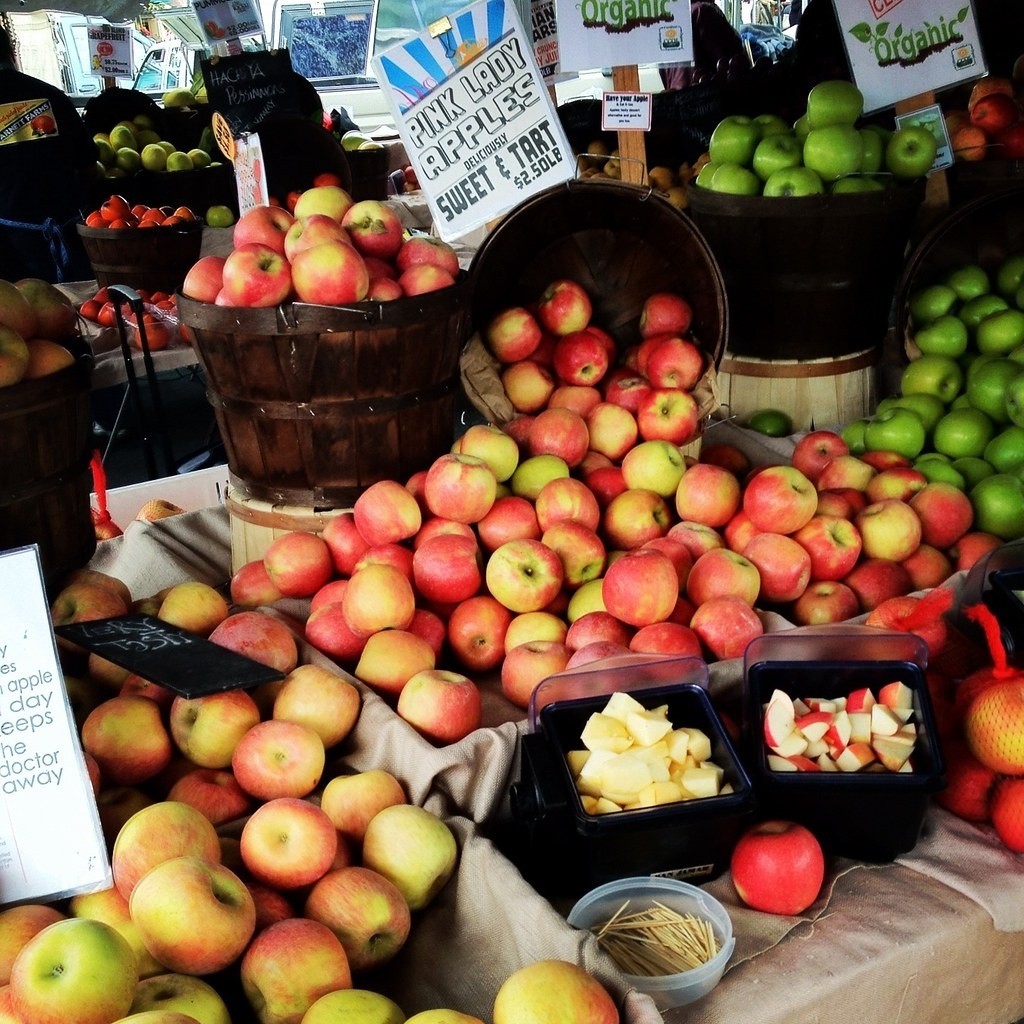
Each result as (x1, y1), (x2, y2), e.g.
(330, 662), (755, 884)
(0, 149), (1024, 584)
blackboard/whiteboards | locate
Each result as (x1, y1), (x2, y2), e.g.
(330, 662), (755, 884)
(200, 48), (293, 115)
(55, 611), (288, 700)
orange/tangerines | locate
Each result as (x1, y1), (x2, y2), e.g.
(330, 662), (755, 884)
(65, 86), (381, 233)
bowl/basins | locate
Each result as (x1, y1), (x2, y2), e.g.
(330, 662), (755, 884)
(747, 660), (948, 857)
(567, 877), (736, 1005)
(536, 680), (754, 855)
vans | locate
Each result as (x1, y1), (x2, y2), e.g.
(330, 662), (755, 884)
(9, 9), (155, 96)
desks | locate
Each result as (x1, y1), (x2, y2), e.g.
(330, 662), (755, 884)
(8, 341), (1024, 1024)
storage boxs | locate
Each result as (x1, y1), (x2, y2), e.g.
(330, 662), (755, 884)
(513, 682), (755, 895)
(743, 654), (942, 857)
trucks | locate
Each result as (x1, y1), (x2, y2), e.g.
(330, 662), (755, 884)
(269, 0), (666, 141)
(130, 38), (265, 107)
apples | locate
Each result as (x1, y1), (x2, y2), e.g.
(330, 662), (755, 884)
(698, 69), (1024, 199)
(0, 185), (1024, 1024)
(574, 141), (711, 209)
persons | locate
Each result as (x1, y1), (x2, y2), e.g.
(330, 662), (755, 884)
(658, 0), (750, 88)
(766, 0), (1024, 129)
(0, 27), (84, 284)
